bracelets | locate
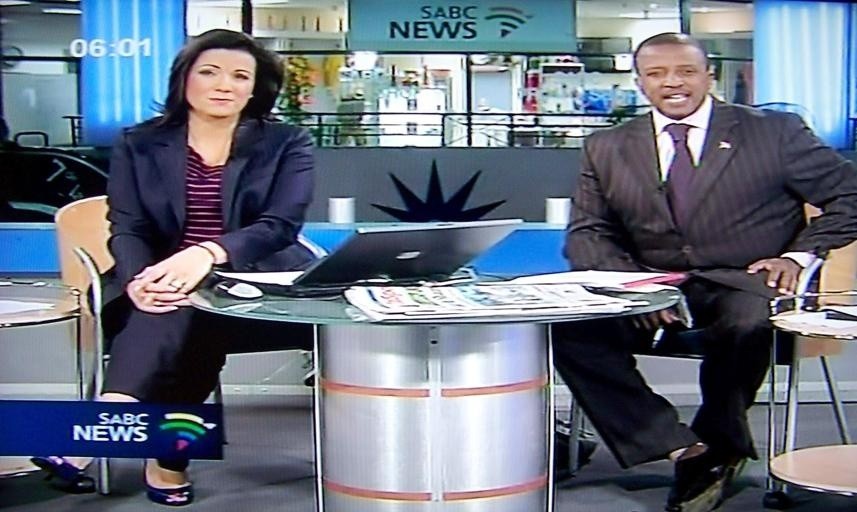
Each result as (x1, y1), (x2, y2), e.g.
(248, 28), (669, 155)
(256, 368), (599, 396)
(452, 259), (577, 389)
(191, 241), (217, 264)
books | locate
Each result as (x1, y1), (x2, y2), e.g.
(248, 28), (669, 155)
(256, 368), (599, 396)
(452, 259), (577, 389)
(336, 268), (689, 330)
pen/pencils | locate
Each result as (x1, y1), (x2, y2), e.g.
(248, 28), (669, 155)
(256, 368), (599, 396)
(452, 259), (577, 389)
(650, 308), (677, 349)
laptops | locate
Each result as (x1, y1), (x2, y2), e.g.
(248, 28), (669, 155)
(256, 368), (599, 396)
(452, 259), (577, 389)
(226, 218), (525, 296)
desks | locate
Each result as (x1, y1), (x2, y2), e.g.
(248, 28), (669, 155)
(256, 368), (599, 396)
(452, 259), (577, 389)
(763, 291), (855, 509)
(188, 273), (683, 512)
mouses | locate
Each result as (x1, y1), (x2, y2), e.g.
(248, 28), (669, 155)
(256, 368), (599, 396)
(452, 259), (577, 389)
(214, 281), (263, 297)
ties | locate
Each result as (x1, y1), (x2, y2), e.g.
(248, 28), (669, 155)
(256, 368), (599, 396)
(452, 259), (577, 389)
(662, 123), (696, 226)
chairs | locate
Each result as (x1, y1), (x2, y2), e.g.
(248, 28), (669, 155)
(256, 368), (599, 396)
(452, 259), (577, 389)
(54, 195), (224, 492)
(563, 203), (856, 494)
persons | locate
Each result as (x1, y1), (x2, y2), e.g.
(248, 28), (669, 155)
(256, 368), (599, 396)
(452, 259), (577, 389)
(24, 27), (318, 507)
(547, 32), (856, 512)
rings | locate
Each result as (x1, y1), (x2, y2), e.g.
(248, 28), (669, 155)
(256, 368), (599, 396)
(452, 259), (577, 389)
(169, 281), (183, 288)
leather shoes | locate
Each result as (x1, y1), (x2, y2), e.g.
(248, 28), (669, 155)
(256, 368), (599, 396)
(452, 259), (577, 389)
(665, 433), (749, 512)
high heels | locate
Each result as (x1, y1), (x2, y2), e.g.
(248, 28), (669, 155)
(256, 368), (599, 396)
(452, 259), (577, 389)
(32, 456), (86, 485)
(141, 462), (195, 508)
